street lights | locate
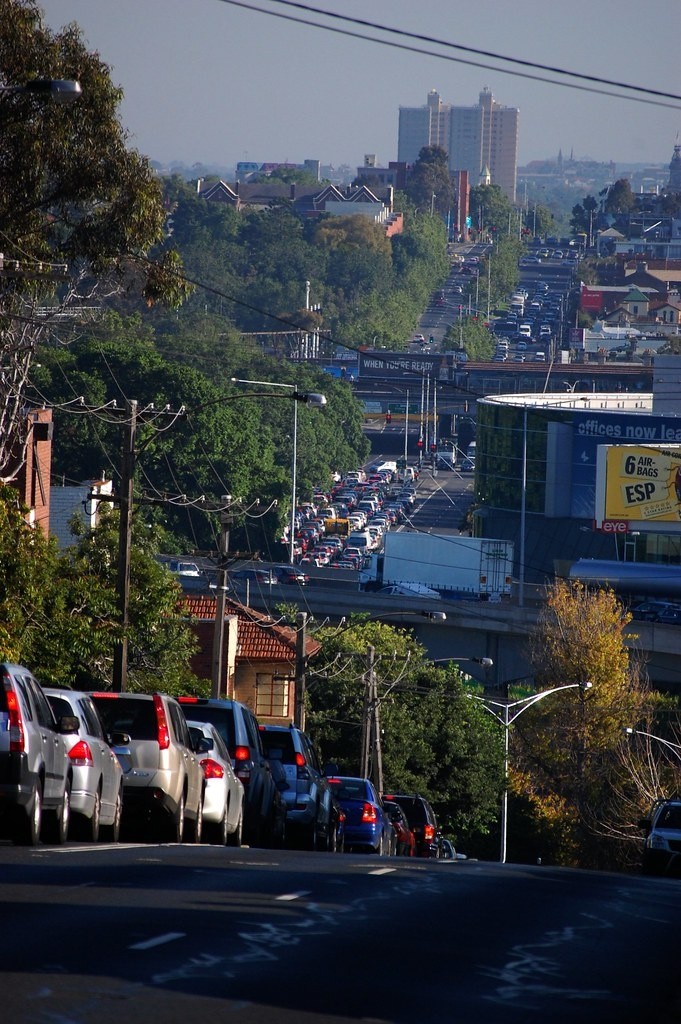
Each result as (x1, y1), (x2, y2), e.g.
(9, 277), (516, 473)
(111, 392), (327, 692)
(359, 657), (494, 780)
(374, 383), (409, 462)
(500, 683), (592, 864)
(295, 610), (420, 741)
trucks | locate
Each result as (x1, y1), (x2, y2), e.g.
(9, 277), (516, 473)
(637, 798), (681, 872)
(357, 531), (514, 604)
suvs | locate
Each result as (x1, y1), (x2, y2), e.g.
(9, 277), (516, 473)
(81, 690), (211, 843)
(257, 722), (339, 851)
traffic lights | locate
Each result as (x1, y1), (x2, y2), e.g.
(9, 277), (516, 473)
(418, 442), (423, 449)
(430, 445), (437, 453)
(386, 414), (391, 423)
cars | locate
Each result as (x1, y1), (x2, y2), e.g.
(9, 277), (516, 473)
(323, 775), (466, 860)
(273, 245), (579, 574)
(41, 687), (132, 844)
(173, 695), (281, 850)
(158, 557), (444, 601)
(0, 660), (81, 846)
(186, 720), (246, 847)
(629, 600), (681, 624)
